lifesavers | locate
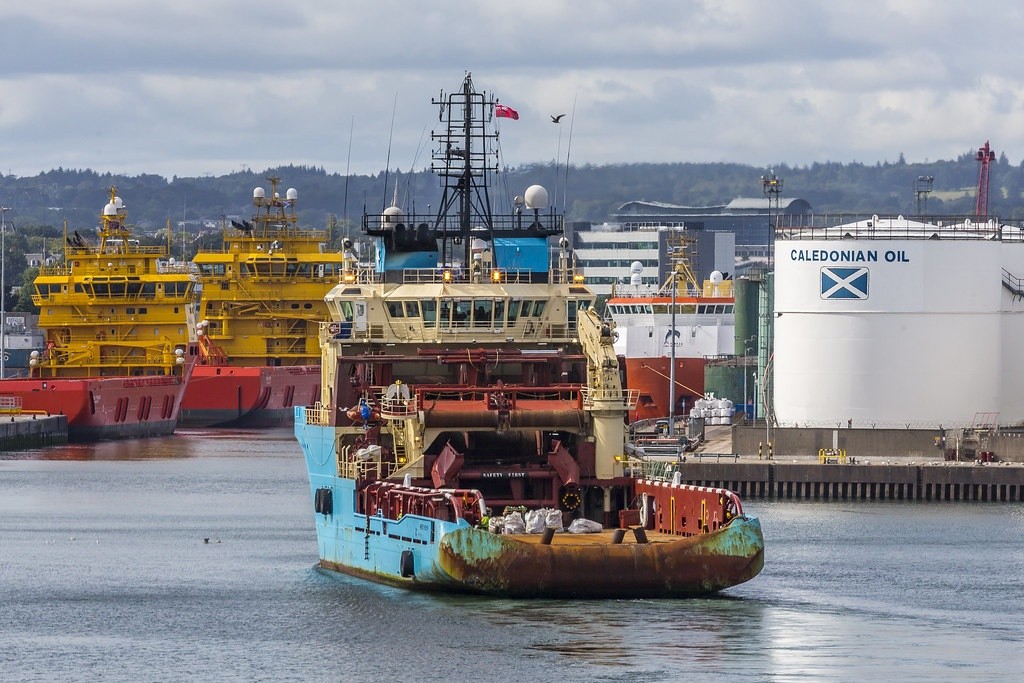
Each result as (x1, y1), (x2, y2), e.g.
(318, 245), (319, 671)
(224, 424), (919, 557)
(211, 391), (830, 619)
(327, 322), (341, 334)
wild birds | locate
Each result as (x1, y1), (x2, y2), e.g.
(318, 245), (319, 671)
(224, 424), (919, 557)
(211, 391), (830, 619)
(550, 114), (566, 123)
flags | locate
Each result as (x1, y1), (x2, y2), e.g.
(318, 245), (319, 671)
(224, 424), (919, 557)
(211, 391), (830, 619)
(496, 104), (518, 120)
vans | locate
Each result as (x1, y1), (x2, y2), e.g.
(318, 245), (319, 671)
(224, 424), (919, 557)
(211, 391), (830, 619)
(654, 420), (669, 430)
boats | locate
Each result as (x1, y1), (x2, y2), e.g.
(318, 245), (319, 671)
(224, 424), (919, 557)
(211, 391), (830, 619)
(294, 69), (766, 597)
(0, 181), (203, 440)
(604, 230), (737, 427)
(174, 176), (354, 431)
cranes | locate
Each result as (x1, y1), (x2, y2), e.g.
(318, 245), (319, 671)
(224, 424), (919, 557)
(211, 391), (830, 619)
(975, 141), (998, 220)
